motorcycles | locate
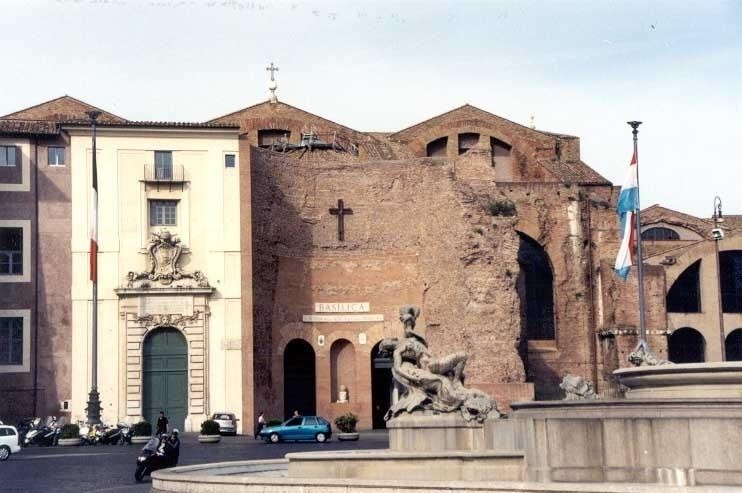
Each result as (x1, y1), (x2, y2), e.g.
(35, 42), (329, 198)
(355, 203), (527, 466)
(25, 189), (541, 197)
(19, 416), (65, 446)
(80, 423), (135, 446)
(135, 438), (177, 482)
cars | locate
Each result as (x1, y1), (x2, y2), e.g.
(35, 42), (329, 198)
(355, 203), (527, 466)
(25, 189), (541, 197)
(0, 425), (21, 461)
(212, 412), (240, 435)
(259, 415), (331, 443)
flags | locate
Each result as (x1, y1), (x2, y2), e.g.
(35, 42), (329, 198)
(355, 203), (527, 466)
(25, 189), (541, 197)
(85, 149), (102, 284)
(608, 151), (651, 281)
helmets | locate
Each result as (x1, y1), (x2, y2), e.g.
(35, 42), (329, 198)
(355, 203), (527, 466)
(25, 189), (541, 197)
(162, 428), (179, 437)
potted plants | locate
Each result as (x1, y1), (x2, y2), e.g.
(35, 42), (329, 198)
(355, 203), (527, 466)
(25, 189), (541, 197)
(335, 411), (362, 440)
(129, 420), (153, 444)
(57, 423), (83, 446)
(198, 420), (221, 443)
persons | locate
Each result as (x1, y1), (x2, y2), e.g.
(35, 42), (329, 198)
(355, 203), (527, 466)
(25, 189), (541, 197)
(254, 411), (266, 440)
(142, 428), (180, 468)
(291, 410), (300, 418)
(378, 313), (470, 391)
(156, 411), (169, 433)
(78, 423), (104, 448)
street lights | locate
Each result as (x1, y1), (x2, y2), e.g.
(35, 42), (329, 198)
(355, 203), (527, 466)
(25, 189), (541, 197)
(712, 197), (726, 362)
